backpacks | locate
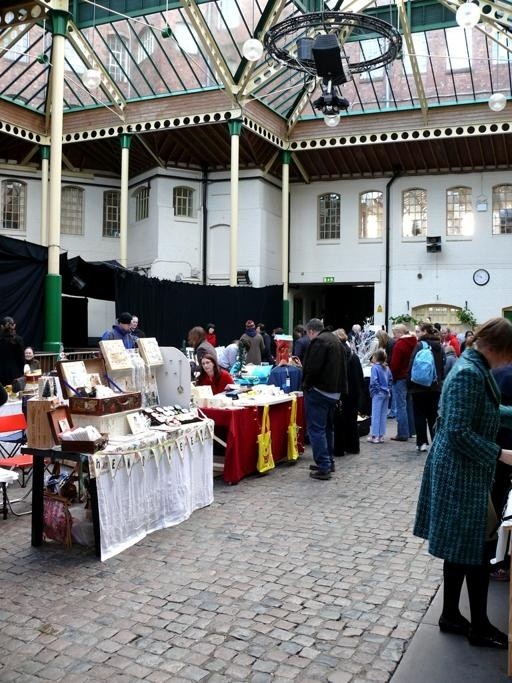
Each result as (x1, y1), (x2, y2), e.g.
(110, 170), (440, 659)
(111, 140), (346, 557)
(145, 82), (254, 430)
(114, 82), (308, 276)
(410, 340), (439, 387)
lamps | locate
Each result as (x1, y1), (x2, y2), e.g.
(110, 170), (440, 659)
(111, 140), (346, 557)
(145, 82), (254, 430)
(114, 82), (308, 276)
(456, 1), (481, 30)
(323, 115), (340, 128)
(82, 0), (100, 90)
(241, 0), (263, 61)
(489, 33), (507, 112)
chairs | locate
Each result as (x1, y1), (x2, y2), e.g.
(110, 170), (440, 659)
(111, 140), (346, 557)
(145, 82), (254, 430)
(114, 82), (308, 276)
(0, 467), (33, 520)
(0, 380), (52, 489)
(0, 414), (52, 517)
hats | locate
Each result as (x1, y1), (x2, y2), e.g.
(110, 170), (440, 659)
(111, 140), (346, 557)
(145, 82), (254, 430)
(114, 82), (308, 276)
(245, 320), (255, 330)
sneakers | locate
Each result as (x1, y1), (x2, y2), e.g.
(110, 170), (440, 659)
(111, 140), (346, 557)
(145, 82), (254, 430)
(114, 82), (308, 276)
(366, 435), (384, 444)
(490, 569), (509, 580)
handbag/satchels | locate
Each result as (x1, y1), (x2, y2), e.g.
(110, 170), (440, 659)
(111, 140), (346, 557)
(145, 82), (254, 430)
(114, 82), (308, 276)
(287, 397), (299, 461)
(256, 405), (275, 473)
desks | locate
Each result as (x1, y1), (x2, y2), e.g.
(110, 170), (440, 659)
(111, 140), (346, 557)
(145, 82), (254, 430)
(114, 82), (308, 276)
(196, 384), (304, 486)
(21, 408), (214, 562)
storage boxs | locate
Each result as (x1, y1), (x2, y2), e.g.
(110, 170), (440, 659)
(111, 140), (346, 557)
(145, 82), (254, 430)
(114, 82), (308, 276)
(69, 407), (142, 439)
(46, 404), (108, 455)
(55, 359), (142, 415)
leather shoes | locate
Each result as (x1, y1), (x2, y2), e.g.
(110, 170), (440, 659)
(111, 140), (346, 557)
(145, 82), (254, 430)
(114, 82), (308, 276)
(309, 464), (335, 472)
(468, 626), (508, 649)
(390, 437), (408, 442)
(438, 615), (471, 637)
(308, 471), (331, 480)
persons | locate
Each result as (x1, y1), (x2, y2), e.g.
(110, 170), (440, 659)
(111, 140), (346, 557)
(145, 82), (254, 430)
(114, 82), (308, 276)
(132, 314), (146, 338)
(367, 349), (393, 445)
(1, 316), (24, 385)
(440, 346), (458, 378)
(294, 323), (311, 356)
(197, 354), (239, 390)
(333, 327), (366, 457)
(304, 318), (348, 480)
(189, 361), (198, 380)
(256, 323), (274, 364)
(188, 326), (217, 381)
(238, 320), (266, 366)
(217, 340), (240, 371)
(102, 312), (138, 347)
(24, 345), (40, 371)
(407, 321), (444, 452)
(434, 316), (473, 355)
(489, 366), (511, 582)
(376, 331), (396, 364)
(415, 319), (512, 682)
(204, 323), (217, 348)
(391, 324), (417, 441)
(272, 327), (285, 337)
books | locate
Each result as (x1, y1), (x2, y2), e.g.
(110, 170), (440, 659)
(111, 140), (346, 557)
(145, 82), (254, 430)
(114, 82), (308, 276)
(59, 361), (92, 398)
(136, 337), (164, 367)
(100, 338), (134, 373)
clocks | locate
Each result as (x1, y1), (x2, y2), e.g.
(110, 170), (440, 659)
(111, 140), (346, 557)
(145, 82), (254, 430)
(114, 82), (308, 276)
(473, 269), (490, 286)
